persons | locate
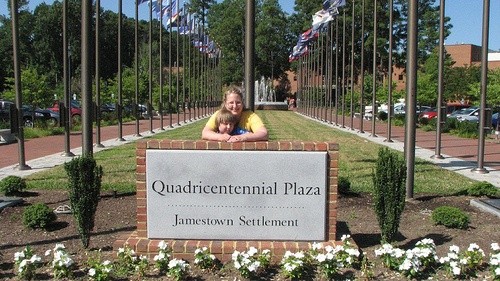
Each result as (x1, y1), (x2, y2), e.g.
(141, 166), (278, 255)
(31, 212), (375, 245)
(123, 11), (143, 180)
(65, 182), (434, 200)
(201, 87), (268, 142)
(215, 110), (253, 134)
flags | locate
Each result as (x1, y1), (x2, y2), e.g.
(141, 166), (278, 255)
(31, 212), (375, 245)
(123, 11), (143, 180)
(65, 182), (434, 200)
(135, 0), (220, 58)
(289, 0), (347, 62)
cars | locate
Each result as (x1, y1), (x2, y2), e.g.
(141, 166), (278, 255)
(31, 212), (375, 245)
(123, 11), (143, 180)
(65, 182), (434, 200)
(418, 108), (439, 124)
(0, 100), (51, 130)
(36, 107), (60, 128)
(101, 98), (147, 116)
(48, 102), (82, 122)
(363, 101), (430, 117)
(447, 106), (499, 130)
